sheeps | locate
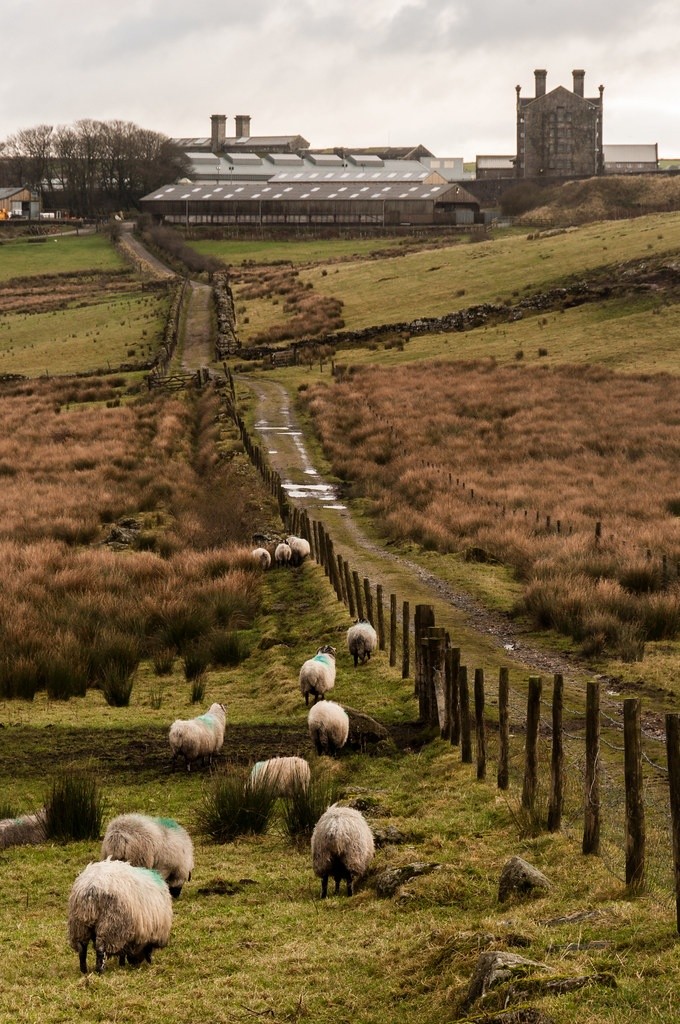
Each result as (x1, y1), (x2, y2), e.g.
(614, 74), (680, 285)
(347, 617), (378, 667)
(308, 699), (350, 758)
(242, 756), (311, 805)
(168, 702), (228, 777)
(68, 855), (174, 975)
(299, 645), (338, 707)
(100, 813), (193, 900)
(252, 536), (311, 574)
(311, 803), (375, 899)
